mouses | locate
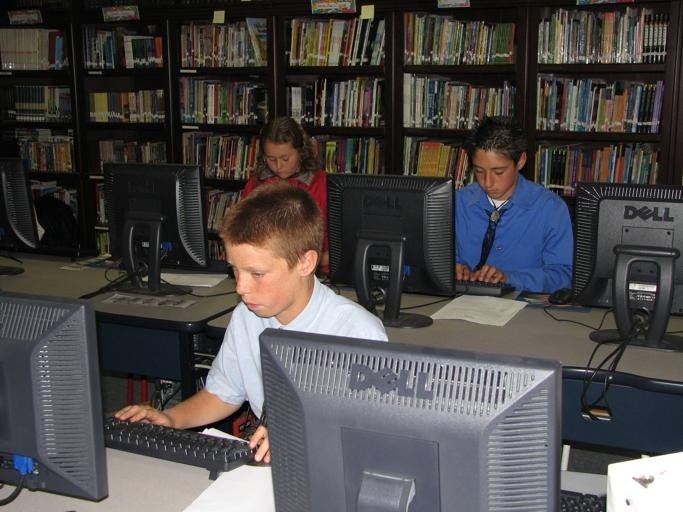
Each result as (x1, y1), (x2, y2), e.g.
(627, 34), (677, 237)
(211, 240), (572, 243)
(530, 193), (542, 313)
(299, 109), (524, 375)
(548, 287), (572, 304)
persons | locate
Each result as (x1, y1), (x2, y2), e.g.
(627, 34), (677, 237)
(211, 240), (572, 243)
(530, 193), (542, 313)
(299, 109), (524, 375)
(109, 183), (388, 467)
(446, 115), (574, 296)
(239, 117), (334, 282)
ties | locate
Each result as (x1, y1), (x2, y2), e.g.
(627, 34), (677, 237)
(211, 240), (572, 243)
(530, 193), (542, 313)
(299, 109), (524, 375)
(475, 209), (504, 269)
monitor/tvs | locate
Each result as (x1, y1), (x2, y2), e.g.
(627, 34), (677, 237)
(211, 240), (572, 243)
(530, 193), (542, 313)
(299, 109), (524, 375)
(1, 295), (111, 501)
(257, 328), (564, 512)
(0, 140), (39, 275)
(572, 180), (682, 350)
(102, 162), (205, 295)
(326, 174), (455, 327)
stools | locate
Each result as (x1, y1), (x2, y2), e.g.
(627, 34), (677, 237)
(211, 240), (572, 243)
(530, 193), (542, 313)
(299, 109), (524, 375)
(116, 367), (179, 412)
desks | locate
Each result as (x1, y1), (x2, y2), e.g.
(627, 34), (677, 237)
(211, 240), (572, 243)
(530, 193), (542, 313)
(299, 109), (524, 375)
(208, 272), (683, 471)
(0, 252), (244, 423)
(0, 418), (278, 512)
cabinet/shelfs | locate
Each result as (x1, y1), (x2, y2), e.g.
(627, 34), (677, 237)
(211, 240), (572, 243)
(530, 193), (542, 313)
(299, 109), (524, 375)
(0, 1), (77, 236)
(169, 0), (278, 266)
(520, 0), (682, 221)
(265, 2), (395, 189)
(65, 3), (179, 252)
(387, 1), (531, 189)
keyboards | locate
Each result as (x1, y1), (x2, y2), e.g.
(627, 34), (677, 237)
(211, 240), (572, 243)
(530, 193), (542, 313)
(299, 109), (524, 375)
(456, 280), (514, 296)
(561, 488), (607, 512)
(105, 417), (253, 480)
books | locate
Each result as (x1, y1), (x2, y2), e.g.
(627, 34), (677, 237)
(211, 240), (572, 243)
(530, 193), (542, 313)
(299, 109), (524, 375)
(0, 2), (263, 260)
(286, 7), (668, 196)
